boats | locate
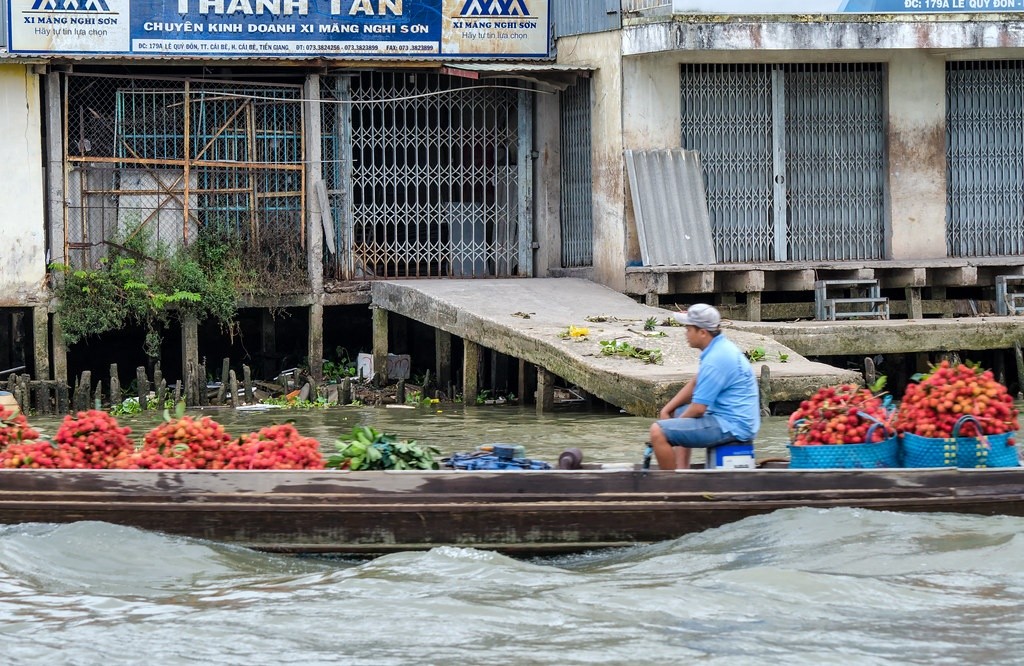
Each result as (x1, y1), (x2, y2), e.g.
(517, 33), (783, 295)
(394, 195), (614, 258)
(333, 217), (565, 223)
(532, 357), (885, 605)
(0, 463), (1024, 564)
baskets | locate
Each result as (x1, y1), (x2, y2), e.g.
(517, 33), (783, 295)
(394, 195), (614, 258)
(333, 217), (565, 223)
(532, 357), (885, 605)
(902, 415), (1020, 468)
(786, 418), (901, 469)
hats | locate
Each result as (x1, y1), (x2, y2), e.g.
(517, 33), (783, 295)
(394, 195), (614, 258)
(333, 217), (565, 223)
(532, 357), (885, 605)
(675, 303), (720, 331)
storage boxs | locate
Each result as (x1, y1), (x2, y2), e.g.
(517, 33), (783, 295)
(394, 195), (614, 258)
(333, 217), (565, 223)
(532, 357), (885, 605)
(705, 444), (754, 469)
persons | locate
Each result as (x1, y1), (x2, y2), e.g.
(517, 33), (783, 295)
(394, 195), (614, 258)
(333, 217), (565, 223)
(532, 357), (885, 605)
(650, 303), (761, 470)
